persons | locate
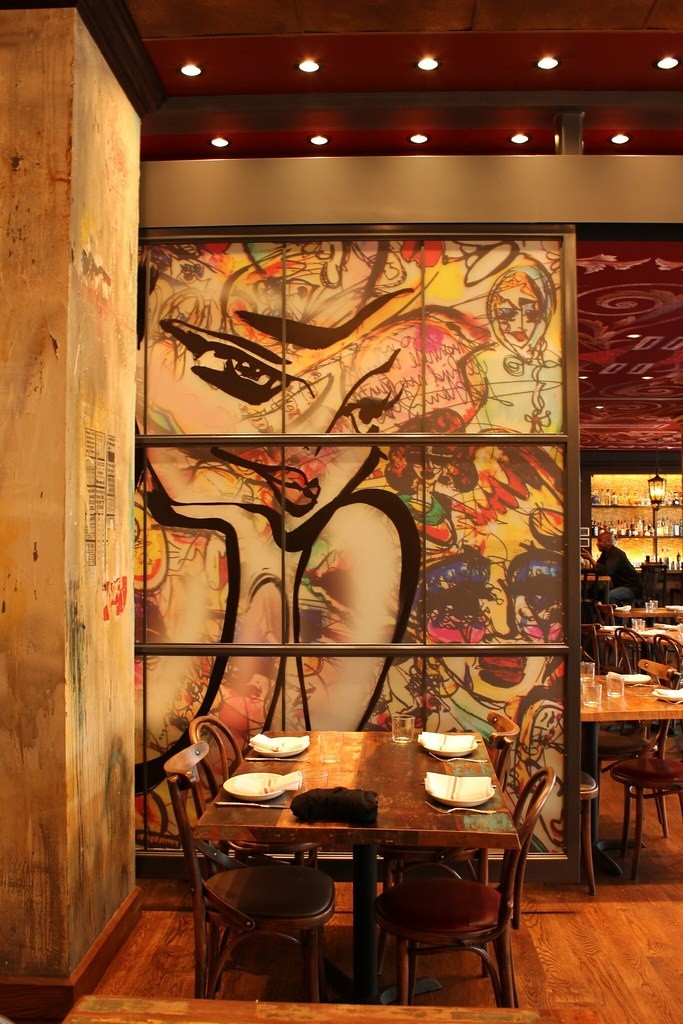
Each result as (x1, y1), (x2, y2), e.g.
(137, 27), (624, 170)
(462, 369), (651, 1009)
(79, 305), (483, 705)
(581, 532), (643, 620)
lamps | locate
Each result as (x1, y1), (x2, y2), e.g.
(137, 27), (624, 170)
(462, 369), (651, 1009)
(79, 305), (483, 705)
(648, 438), (668, 509)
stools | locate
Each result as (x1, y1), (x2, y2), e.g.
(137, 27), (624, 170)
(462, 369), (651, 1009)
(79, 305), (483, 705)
(628, 597), (644, 608)
(581, 569), (599, 626)
(670, 588), (682, 625)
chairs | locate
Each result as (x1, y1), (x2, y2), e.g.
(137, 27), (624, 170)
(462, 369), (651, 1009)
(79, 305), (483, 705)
(165, 603), (683, 1007)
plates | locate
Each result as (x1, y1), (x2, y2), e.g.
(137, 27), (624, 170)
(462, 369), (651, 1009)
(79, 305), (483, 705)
(222, 773), (286, 800)
(423, 741), (478, 758)
(600, 606), (631, 631)
(624, 674), (683, 703)
(430, 785), (495, 806)
(639, 605), (683, 637)
(253, 737), (308, 757)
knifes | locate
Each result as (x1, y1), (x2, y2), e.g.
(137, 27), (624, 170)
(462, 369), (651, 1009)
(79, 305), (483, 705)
(214, 802), (290, 808)
(244, 756), (312, 762)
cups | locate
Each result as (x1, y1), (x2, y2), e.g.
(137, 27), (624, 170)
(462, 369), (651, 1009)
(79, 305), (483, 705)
(317, 731), (344, 763)
(298, 769), (328, 795)
(392, 715), (415, 744)
(580, 662), (595, 685)
(632, 600), (658, 631)
(582, 683), (602, 708)
(606, 676), (624, 697)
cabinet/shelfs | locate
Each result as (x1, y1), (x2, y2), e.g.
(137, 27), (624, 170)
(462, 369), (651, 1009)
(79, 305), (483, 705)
(591, 501), (683, 575)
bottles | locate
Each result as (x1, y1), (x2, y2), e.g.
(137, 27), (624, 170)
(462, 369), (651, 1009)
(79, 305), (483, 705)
(580, 485), (683, 570)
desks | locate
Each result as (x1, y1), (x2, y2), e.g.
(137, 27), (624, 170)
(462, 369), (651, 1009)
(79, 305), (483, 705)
(613, 606), (683, 627)
(581, 673), (683, 881)
(595, 625), (683, 735)
(194, 730), (522, 1007)
(60, 995), (541, 1024)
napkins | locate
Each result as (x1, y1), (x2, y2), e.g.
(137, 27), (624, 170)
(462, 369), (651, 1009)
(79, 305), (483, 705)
(601, 626), (624, 632)
(666, 605), (683, 610)
(640, 630), (666, 635)
(425, 771), (492, 800)
(235, 770), (302, 794)
(616, 605), (632, 610)
(655, 688), (683, 697)
(607, 671), (651, 681)
(248, 733), (310, 753)
(417, 732), (475, 750)
(654, 623), (683, 630)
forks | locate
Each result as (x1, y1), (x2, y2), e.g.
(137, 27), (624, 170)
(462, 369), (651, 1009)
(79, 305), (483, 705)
(424, 800), (496, 814)
(428, 752), (488, 763)
